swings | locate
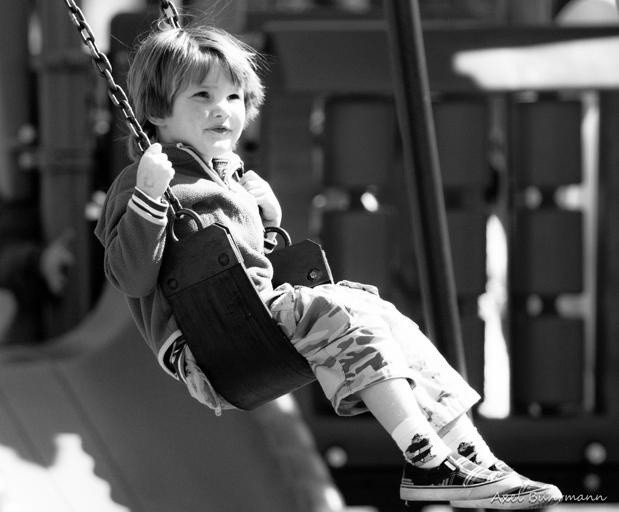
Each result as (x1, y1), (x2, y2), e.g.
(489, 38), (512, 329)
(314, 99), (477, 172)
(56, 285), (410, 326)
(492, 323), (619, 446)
(65, 0), (334, 411)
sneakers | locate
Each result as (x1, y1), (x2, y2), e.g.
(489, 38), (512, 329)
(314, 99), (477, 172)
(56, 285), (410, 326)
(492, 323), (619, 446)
(449, 458), (566, 510)
(398, 451), (522, 502)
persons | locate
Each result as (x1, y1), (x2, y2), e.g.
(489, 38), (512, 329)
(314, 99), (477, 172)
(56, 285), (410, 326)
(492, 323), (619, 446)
(94, 25), (564, 509)
